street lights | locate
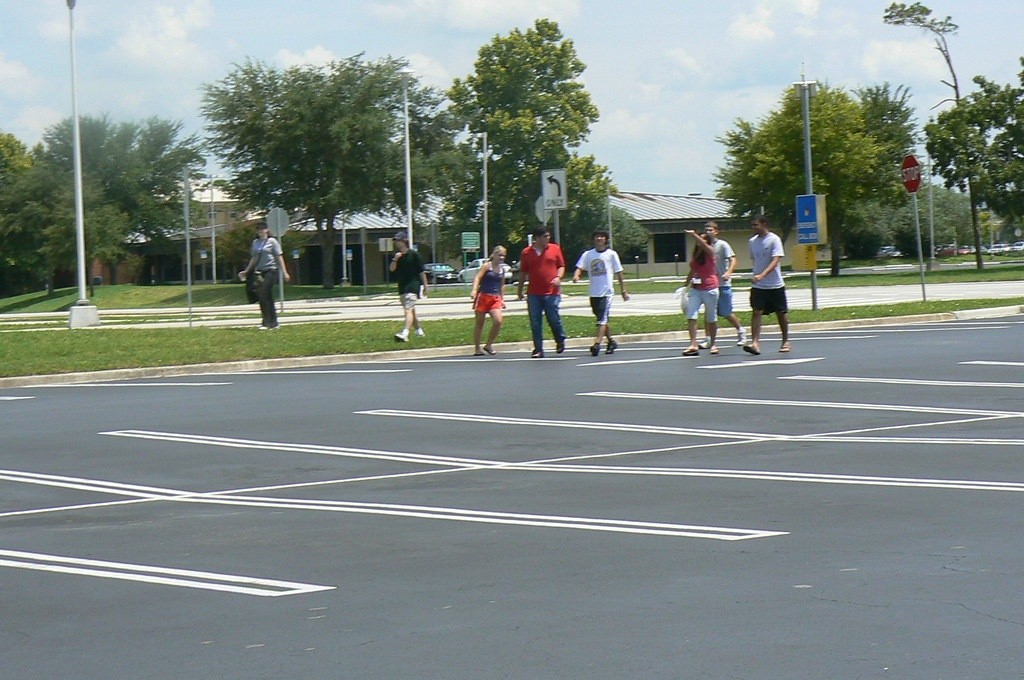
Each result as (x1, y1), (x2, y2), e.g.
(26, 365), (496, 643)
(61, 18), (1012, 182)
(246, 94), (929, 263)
(475, 131), (492, 259)
(793, 60), (825, 309)
(395, 65), (420, 251)
(65, 0), (106, 329)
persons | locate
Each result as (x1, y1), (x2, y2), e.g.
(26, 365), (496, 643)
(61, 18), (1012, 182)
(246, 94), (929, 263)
(390, 232), (428, 342)
(683, 230), (719, 356)
(572, 230), (629, 356)
(241, 222), (290, 330)
(518, 227), (566, 357)
(743, 215), (791, 355)
(470, 246), (507, 355)
(698, 222), (746, 349)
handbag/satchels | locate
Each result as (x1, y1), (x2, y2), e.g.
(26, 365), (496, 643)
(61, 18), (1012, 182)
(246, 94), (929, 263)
(246, 268), (260, 303)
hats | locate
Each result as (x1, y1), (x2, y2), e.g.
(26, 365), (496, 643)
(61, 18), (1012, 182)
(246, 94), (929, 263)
(391, 231), (408, 241)
(254, 222), (268, 231)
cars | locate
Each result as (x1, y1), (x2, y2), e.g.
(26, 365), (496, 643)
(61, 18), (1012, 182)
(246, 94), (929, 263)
(936, 240), (1024, 258)
(876, 247), (903, 260)
(456, 258), (513, 285)
(421, 263), (460, 284)
(512, 261), (531, 282)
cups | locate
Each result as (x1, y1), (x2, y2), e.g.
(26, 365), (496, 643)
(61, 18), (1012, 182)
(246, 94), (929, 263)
(239, 272), (247, 282)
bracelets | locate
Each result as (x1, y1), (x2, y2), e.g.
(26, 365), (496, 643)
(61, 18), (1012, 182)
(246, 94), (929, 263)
(621, 289), (626, 294)
(556, 276), (562, 280)
(392, 258), (398, 262)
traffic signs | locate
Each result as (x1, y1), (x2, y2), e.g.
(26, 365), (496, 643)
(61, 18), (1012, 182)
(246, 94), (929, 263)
(541, 169), (569, 212)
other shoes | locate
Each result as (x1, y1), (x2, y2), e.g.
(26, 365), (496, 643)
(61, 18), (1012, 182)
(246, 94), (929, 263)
(698, 341), (711, 349)
(710, 346), (718, 354)
(590, 344), (600, 356)
(779, 346), (791, 352)
(394, 333), (408, 342)
(474, 351), (484, 355)
(743, 345), (760, 355)
(604, 339), (618, 354)
(533, 351), (542, 358)
(682, 347), (698, 356)
(556, 341), (564, 353)
(484, 347), (496, 355)
(737, 338), (747, 345)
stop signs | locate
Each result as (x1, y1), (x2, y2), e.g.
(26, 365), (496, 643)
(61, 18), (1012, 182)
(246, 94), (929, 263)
(900, 154), (924, 192)
